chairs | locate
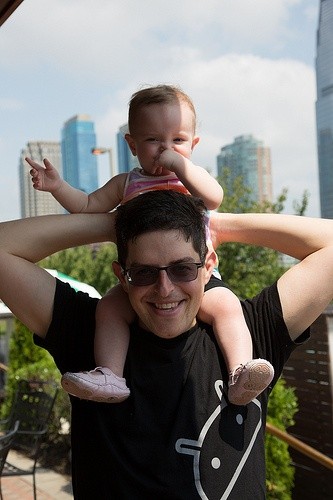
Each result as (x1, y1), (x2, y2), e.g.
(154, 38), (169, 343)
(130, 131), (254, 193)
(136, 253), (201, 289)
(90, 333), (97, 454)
(0, 420), (19, 500)
(1, 379), (60, 500)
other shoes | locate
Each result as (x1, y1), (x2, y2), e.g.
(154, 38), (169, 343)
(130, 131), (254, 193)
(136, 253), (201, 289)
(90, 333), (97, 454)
(228, 358), (275, 405)
(61, 366), (130, 403)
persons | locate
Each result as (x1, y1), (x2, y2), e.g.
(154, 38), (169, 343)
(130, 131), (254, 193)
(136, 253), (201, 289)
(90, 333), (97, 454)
(25, 84), (274, 406)
(0, 189), (333, 500)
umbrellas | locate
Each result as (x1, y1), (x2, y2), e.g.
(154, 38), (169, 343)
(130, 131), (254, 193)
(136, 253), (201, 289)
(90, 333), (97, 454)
(0, 268), (103, 355)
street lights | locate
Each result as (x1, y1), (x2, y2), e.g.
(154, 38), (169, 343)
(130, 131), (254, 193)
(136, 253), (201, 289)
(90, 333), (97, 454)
(91, 146), (115, 177)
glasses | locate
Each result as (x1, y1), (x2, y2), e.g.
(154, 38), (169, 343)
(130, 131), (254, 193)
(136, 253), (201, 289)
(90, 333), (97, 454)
(120, 259), (205, 287)
